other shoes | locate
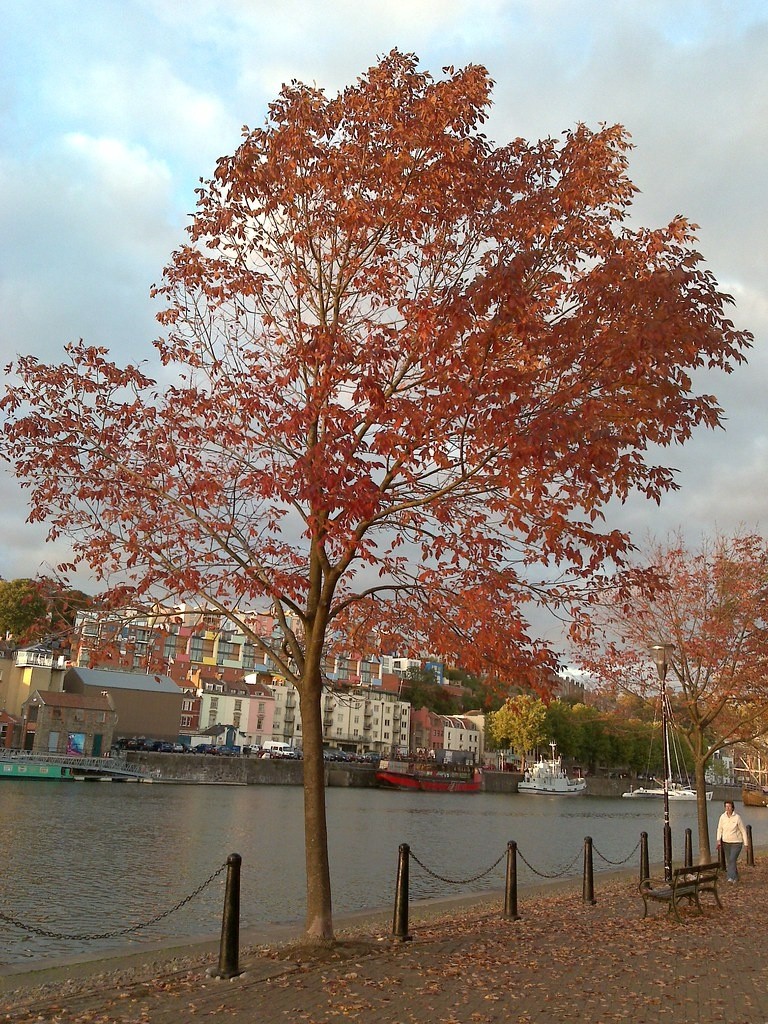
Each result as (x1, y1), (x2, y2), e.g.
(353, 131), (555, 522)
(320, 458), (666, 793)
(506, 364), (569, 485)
(728, 878), (733, 882)
(732, 879), (738, 884)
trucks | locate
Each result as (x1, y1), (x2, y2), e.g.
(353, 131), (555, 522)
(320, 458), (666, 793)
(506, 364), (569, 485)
(263, 741), (295, 756)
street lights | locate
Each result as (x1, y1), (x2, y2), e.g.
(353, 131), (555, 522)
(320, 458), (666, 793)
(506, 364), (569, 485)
(648, 643), (682, 880)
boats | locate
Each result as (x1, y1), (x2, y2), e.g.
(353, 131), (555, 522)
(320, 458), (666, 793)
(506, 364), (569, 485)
(517, 740), (587, 796)
(376, 759), (483, 793)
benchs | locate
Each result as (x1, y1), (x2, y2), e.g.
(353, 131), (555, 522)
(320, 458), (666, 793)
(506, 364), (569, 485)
(638, 862), (724, 924)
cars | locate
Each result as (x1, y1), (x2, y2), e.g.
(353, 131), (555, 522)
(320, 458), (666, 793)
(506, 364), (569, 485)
(362, 754), (373, 763)
(363, 752), (379, 760)
(500, 762), (518, 772)
(115, 739), (140, 750)
(324, 747), (348, 761)
(323, 750), (329, 760)
(153, 741), (173, 753)
(347, 752), (364, 763)
(217, 746), (232, 755)
(194, 744), (217, 755)
(483, 764), (496, 770)
(183, 744), (197, 753)
(249, 744), (261, 753)
(172, 743), (184, 753)
(135, 739), (156, 751)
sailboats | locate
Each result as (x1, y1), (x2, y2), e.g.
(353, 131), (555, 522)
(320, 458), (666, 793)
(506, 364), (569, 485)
(622, 679), (713, 802)
(733, 751), (768, 806)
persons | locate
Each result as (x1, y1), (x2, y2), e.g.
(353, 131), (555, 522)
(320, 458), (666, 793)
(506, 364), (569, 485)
(717, 801), (748, 883)
